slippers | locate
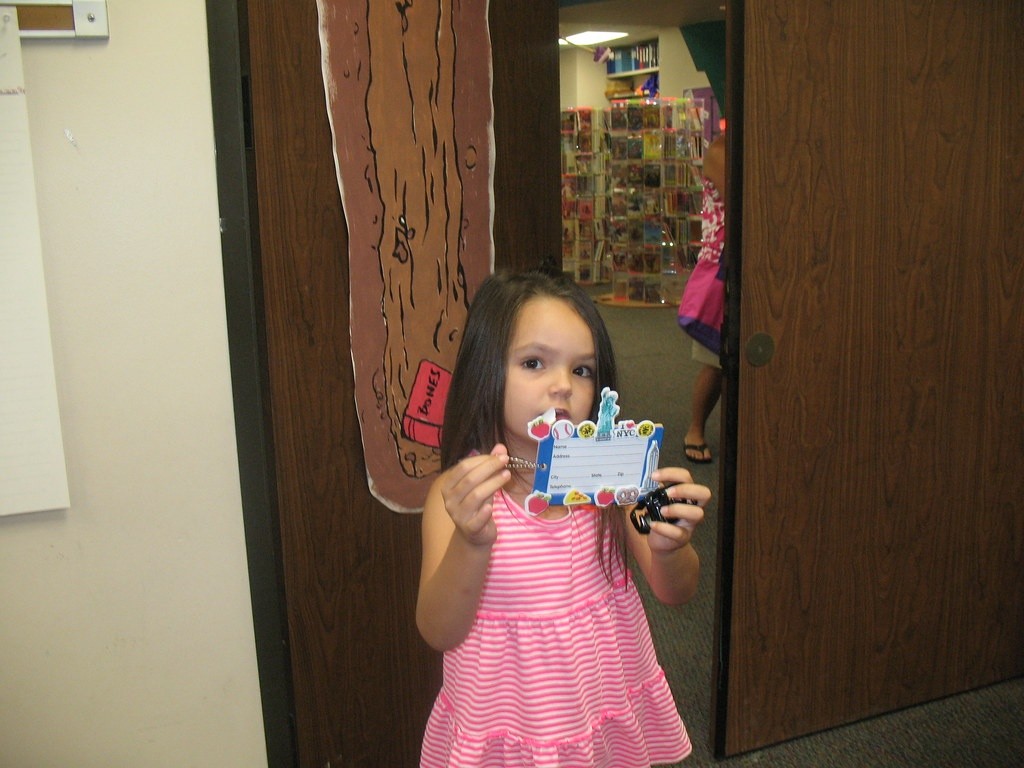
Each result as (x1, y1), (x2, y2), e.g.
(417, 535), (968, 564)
(683, 438), (711, 463)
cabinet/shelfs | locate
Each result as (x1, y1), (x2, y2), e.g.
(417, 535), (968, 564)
(605, 66), (659, 98)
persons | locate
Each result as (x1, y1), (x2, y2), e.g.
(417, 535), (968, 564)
(410, 268), (715, 766)
(677, 135), (728, 466)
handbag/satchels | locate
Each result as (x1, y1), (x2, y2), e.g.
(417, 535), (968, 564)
(677, 254), (722, 362)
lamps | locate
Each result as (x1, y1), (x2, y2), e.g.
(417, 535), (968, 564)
(559, 33), (611, 64)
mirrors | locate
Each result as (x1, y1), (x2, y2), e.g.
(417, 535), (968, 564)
(555, 0), (731, 765)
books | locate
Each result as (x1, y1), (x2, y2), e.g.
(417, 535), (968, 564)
(558, 43), (707, 309)
